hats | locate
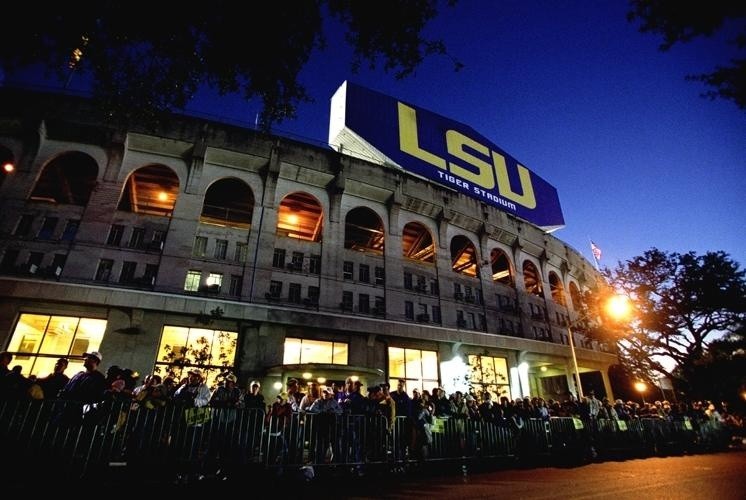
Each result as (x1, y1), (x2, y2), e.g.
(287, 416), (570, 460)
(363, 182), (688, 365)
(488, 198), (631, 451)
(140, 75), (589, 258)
(224, 374), (237, 383)
(187, 369), (202, 376)
(323, 387), (335, 395)
(399, 379), (531, 406)
(83, 351), (102, 361)
(251, 380), (260, 387)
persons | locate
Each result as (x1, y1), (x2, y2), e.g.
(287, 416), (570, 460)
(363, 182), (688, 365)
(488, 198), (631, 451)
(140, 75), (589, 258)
(1, 350), (746, 500)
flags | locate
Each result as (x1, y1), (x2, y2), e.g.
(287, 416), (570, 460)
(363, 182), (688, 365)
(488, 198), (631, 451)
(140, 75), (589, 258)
(590, 241), (602, 261)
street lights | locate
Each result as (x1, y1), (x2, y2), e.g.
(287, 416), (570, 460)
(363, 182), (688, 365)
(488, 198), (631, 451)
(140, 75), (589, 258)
(635, 382), (647, 403)
(565, 296), (627, 404)
